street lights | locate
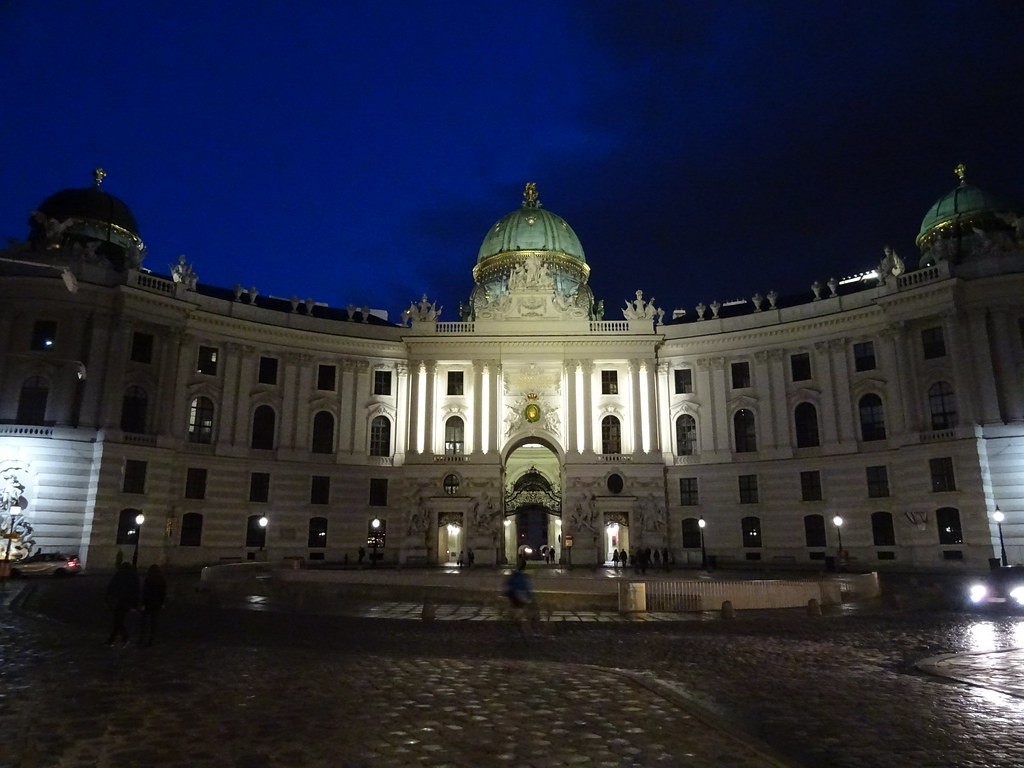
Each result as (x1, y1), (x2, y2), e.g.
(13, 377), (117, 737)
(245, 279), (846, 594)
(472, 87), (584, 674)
(3, 499), (21, 559)
(372, 515), (381, 566)
(259, 512), (268, 551)
(991, 503), (1009, 568)
(832, 511), (843, 556)
(694, 513), (708, 567)
(129, 510), (144, 569)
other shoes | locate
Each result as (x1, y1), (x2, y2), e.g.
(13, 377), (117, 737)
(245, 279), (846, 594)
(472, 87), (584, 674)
(145, 640), (154, 647)
(122, 640), (130, 649)
(104, 642), (115, 648)
(136, 640), (144, 646)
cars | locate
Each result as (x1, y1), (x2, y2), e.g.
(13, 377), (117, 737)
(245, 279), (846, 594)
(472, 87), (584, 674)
(9, 551), (82, 581)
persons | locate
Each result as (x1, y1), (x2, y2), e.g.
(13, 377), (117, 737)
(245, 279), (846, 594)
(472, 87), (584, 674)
(641, 494), (660, 530)
(538, 264), (550, 278)
(140, 565), (168, 644)
(576, 490), (593, 531)
(619, 549), (627, 568)
(467, 548), (474, 568)
(473, 494), (492, 532)
(549, 547), (555, 564)
(408, 495), (428, 535)
(631, 547), (669, 574)
(418, 295), (431, 314)
(507, 559), (539, 645)
(457, 550), (464, 567)
(174, 260), (188, 284)
(634, 291), (646, 318)
(525, 252), (541, 283)
(513, 263), (525, 275)
(104, 561), (141, 648)
(612, 549), (619, 568)
(545, 548), (550, 563)
